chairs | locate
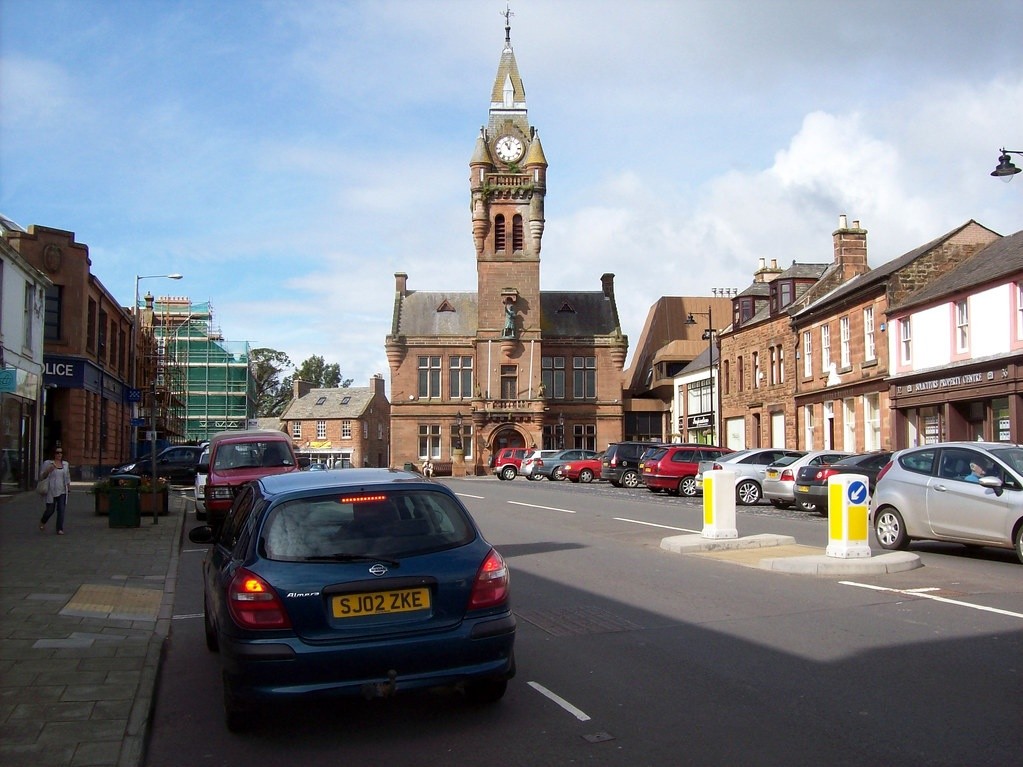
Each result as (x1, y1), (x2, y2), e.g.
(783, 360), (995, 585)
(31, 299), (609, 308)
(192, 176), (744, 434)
(352, 501), (400, 525)
(953, 459), (970, 480)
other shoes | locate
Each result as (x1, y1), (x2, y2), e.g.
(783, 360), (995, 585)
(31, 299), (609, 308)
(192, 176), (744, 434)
(39, 521), (45, 531)
(57, 530), (64, 536)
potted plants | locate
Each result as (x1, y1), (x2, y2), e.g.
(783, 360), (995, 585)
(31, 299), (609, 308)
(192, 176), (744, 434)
(89, 475), (169, 517)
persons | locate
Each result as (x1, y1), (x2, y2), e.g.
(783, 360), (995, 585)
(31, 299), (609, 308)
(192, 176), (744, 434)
(39, 447), (71, 535)
(964, 455), (988, 485)
(503, 304), (516, 338)
(421, 459), (434, 478)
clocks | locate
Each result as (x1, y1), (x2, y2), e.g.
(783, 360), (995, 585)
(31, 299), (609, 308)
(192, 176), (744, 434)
(494, 133), (524, 164)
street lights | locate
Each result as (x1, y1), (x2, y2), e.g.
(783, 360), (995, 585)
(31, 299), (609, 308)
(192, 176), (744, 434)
(683, 308), (715, 448)
(134, 273), (184, 459)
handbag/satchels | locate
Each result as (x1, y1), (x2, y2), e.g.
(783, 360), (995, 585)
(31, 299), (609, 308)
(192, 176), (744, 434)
(35, 478), (48, 495)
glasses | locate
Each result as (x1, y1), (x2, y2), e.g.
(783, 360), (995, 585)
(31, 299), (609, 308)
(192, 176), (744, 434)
(54, 451), (64, 454)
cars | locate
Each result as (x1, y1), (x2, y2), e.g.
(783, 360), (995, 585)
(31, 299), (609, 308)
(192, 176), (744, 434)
(694, 449), (793, 507)
(204, 428), (303, 534)
(111, 445), (203, 486)
(761, 452), (855, 513)
(492, 441), (737, 497)
(870, 440), (1023, 564)
(793, 450), (894, 520)
(193, 448), (210, 521)
(189, 466), (517, 733)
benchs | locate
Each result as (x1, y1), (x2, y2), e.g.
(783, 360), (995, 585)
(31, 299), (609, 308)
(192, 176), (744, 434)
(287, 519), (429, 542)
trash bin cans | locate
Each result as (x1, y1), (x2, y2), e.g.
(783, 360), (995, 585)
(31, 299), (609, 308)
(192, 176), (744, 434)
(404, 462), (414, 471)
(104, 475), (142, 529)
